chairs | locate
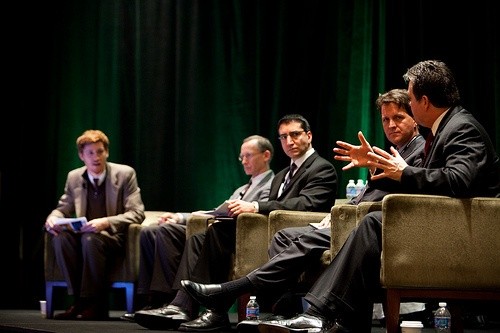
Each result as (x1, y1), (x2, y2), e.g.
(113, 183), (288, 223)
(47, 194), (499, 333)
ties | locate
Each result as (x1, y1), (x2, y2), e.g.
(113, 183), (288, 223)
(93, 178), (99, 188)
(282, 164), (297, 191)
(240, 180), (252, 200)
(422, 130), (433, 167)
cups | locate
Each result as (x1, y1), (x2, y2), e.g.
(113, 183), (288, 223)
(39, 301), (47, 315)
(400, 320), (424, 333)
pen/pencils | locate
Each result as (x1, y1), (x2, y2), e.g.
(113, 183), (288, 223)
(158, 215), (176, 223)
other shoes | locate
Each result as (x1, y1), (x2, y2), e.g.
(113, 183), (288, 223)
(76, 307), (109, 321)
(55, 305), (81, 320)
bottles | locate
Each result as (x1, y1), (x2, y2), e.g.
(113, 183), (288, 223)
(355, 179), (364, 194)
(346, 179), (357, 202)
(246, 296), (259, 321)
(434, 302), (452, 333)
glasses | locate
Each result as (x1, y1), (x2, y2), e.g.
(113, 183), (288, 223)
(239, 151), (265, 161)
(278, 131), (305, 141)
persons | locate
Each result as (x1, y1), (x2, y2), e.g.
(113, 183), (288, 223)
(46, 130), (145, 322)
(236, 59), (500, 333)
(135, 112), (339, 331)
(122, 135), (275, 319)
(180, 89), (426, 333)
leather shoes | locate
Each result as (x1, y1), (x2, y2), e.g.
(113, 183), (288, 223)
(179, 310), (231, 332)
(258, 314), (340, 333)
(121, 306), (151, 320)
(180, 279), (233, 314)
(134, 305), (189, 330)
